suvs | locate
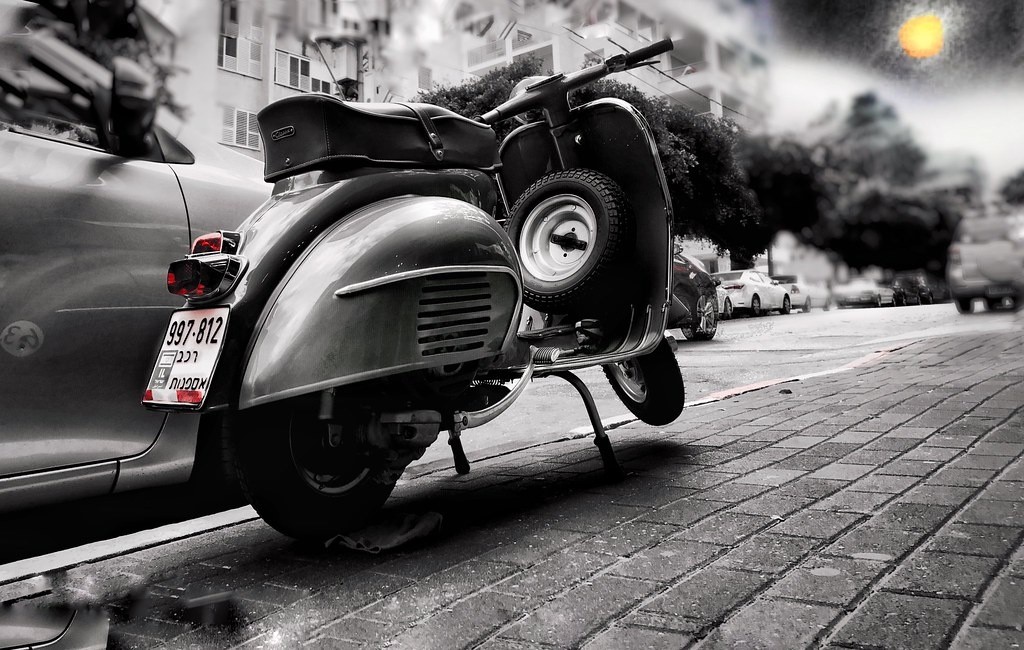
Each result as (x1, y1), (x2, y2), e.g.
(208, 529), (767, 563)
(948, 204), (1024, 313)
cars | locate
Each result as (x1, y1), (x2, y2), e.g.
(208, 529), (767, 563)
(0, 0), (276, 505)
(710, 269), (792, 321)
(891, 274), (935, 307)
(771, 273), (833, 313)
(664, 246), (719, 342)
(833, 278), (895, 307)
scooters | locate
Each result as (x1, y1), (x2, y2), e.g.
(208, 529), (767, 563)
(143, 35), (686, 550)
